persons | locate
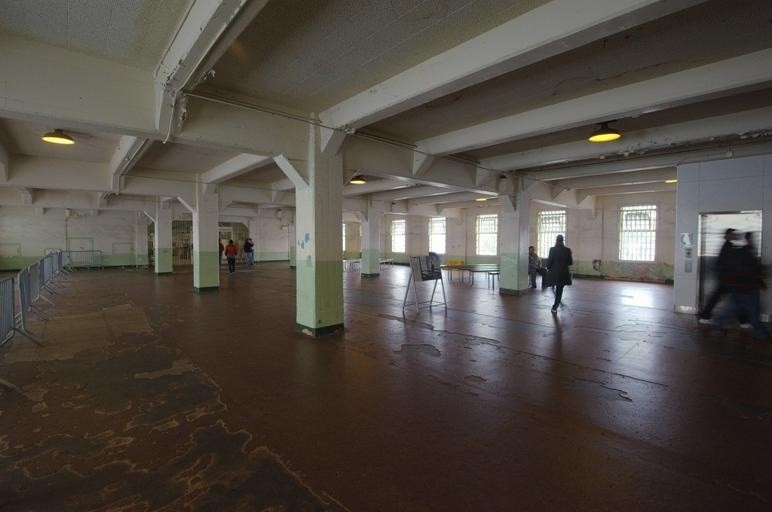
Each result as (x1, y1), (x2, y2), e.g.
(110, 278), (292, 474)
(527, 244), (547, 290)
(243, 239), (252, 266)
(248, 239), (256, 265)
(545, 235), (574, 313)
(219, 240), (224, 270)
(225, 240), (239, 273)
(695, 228), (755, 330)
(429, 261), (436, 276)
(712, 231), (770, 339)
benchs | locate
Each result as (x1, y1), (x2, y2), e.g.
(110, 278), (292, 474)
(439, 260), (501, 292)
(343, 256), (395, 273)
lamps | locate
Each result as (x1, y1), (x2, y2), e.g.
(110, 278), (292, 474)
(584, 119), (625, 144)
(40, 127), (76, 146)
(350, 174), (369, 185)
